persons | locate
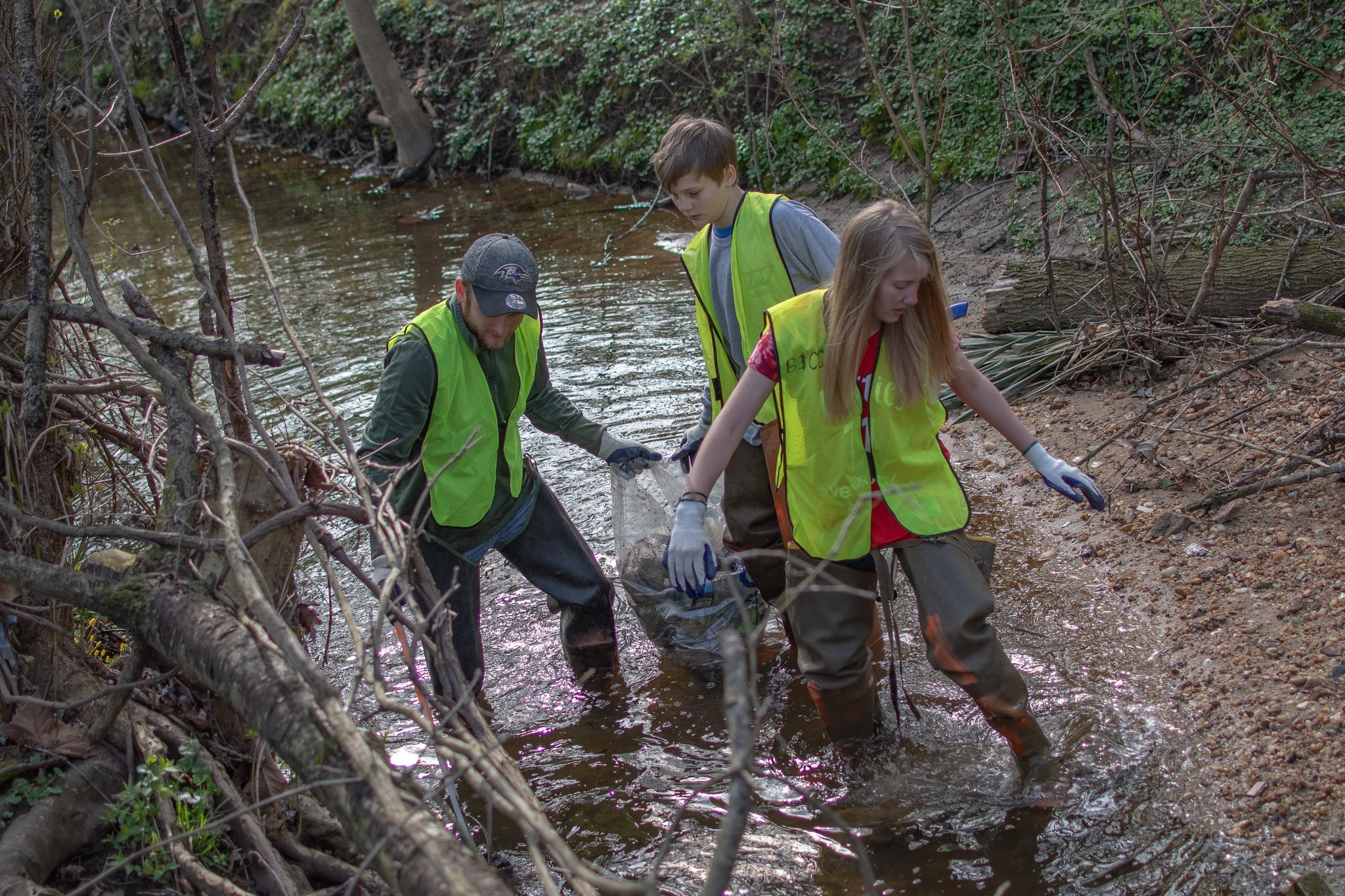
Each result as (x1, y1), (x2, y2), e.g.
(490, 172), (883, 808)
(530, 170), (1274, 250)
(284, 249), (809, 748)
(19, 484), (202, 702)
(653, 118), (884, 650)
(663, 202), (1105, 767)
(355, 231), (663, 723)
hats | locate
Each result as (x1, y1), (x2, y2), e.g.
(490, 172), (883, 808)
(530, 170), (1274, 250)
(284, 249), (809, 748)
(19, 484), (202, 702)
(461, 232), (540, 319)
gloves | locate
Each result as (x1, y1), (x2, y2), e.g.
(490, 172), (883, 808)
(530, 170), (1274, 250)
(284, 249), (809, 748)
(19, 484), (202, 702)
(596, 430), (663, 478)
(671, 419), (711, 474)
(1020, 438), (1108, 511)
(661, 501), (719, 600)
(372, 552), (407, 622)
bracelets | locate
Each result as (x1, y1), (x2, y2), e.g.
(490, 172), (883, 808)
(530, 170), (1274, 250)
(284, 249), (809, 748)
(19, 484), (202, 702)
(682, 491), (707, 502)
